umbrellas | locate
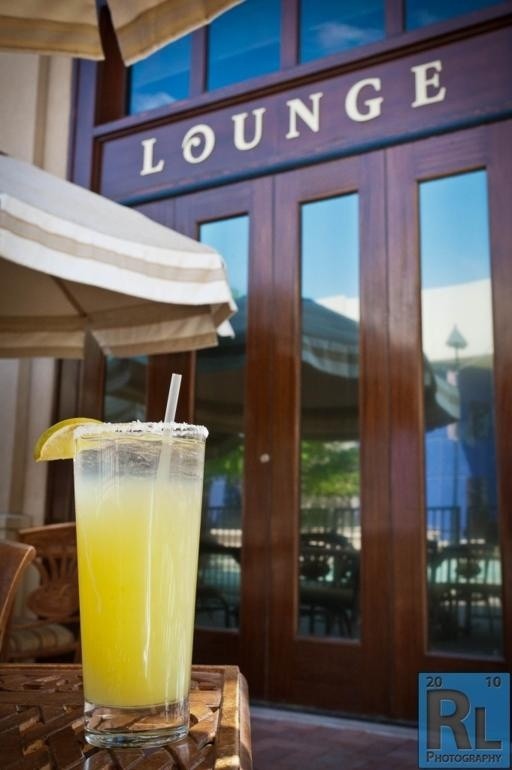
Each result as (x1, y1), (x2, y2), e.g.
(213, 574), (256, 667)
(0, 0), (256, 83)
(0, 146), (239, 362)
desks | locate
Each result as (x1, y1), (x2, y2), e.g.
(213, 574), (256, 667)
(2, 663), (251, 770)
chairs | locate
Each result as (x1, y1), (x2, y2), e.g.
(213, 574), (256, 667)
(3, 540), (37, 663)
(7, 520), (82, 658)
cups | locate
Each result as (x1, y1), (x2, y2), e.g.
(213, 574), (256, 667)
(70, 421), (211, 749)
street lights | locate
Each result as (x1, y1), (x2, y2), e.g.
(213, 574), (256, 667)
(444, 319), (466, 538)
